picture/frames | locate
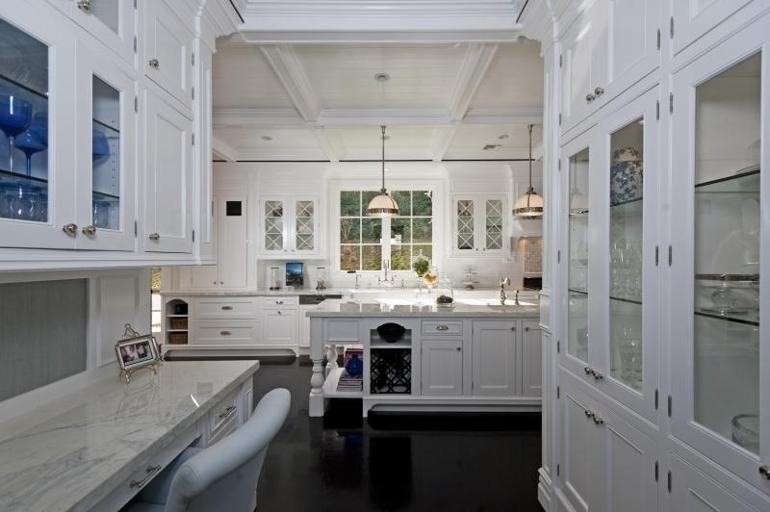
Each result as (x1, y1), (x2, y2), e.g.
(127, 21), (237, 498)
(111, 334), (161, 370)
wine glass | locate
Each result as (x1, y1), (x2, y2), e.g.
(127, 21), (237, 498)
(15, 112), (48, 177)
(425, 267), (439, 296)
(0, 96), (33, 174)
(710, 258), (759, 319)
(569, 242), (642, 388)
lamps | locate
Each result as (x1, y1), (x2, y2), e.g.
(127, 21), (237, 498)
(514, 123), (548, 217)
(568, 149), (591, 217)
(366, 123), (404, 216)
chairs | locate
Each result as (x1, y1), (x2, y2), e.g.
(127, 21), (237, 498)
(140, 388), (297, 512)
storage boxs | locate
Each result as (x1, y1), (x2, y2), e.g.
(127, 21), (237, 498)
(171, 317), (188, 330)
(169, 332), (188, 345)
(174, 304), (188, 315)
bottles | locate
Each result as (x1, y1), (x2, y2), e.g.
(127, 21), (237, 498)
(610, 146), (642, 203)
(435, 273), (454, 307)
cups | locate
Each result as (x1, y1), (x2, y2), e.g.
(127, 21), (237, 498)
(92, 200), (109, 227)
(92, 130), (109, 162)
(0, 182), (48, 229)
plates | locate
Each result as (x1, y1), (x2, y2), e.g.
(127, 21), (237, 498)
(736, 165), (760, 187)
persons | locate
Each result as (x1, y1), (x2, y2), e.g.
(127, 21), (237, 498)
(122, 346), (134, 363)
(136, 343), (148, 358)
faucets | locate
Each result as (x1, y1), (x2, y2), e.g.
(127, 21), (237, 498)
(514, 289), (521, 306)
(497, 276), (511, 306)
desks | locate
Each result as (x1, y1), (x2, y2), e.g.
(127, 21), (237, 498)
(0, 352), (260, 511)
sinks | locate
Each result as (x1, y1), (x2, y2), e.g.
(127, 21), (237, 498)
(452, 296), (540, 306)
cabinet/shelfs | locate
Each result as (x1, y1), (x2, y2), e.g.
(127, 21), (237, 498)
(195, 297), (261, 346)
(256, 182), (327, 259)
(161, 293), (194, 354)
(666, 1), (766, 74)
(419, 318), (464, 396)
(140, 0), (200, 254)
(0, 2), (137, 255)
(559, 0), (662, 133)
(560, 370), (661, 512)
(180, 175), (256, 294)
(469, 318), (545, 399)
(445, 174), (511, 260)
(661, 435), (770, 511)
(261, 297), (297, 345)
(666, 11), (768, 500)
(559, 80), (661, 424)
